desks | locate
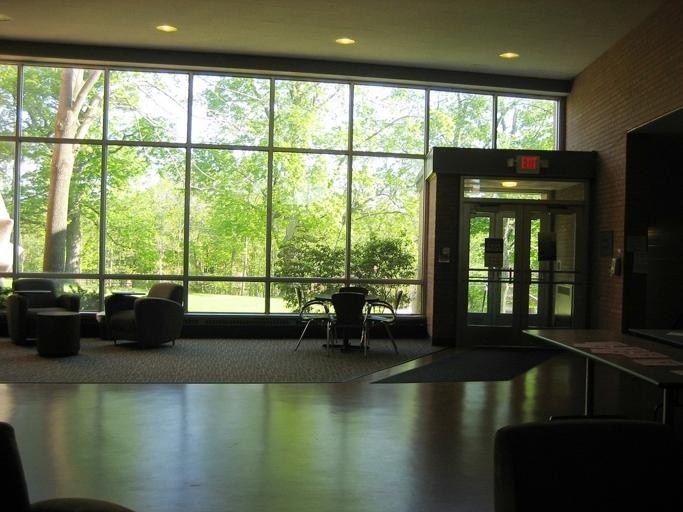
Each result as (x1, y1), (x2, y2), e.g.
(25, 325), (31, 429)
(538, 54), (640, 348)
(519, 326), (683, 426)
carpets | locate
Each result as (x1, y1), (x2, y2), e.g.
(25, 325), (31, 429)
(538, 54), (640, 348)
(1, 338), (449, 383)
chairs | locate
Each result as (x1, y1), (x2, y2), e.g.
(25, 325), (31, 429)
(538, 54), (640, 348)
(294, 286), (401, 361)
(492, 419), (683, 511)
(0, 277), (192, 357)
